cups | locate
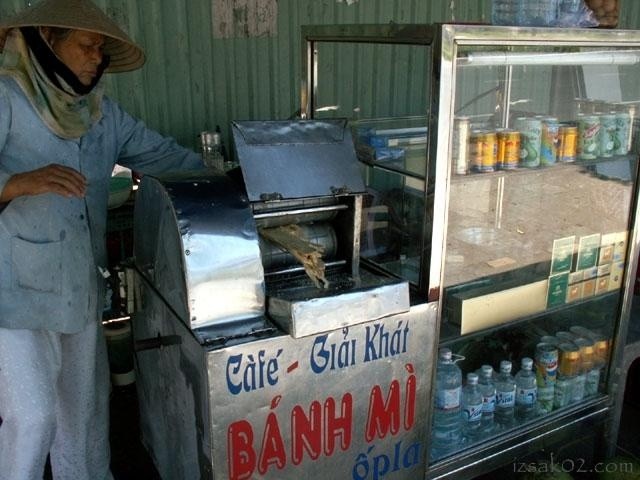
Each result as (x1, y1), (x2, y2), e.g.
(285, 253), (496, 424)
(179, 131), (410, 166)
(201, 132), (222, 168)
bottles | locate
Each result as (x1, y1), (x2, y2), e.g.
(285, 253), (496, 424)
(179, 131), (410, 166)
(426, 346), (539, 460)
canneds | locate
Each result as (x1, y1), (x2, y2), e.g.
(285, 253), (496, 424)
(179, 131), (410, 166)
(600, 113), (615, 159)
(471, 128), (497, 173)
(539, 120), (556, 165)
(533, 325), (609, 416)
(576, 115), (601, 161)
(452, 117), (470, 176)
(495, 127), (520, 170)
(557, 124), (576, 162)
(614, 114), (631, 156)
(514, 117), (541, 168)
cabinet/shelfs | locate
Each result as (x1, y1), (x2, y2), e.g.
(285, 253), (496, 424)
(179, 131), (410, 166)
(302, 21), (640, 480)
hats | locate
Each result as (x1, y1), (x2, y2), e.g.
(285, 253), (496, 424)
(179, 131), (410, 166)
(0, 0), (147, 74)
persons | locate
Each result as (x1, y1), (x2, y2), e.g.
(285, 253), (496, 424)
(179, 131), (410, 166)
(0, 1), (222, 480)
